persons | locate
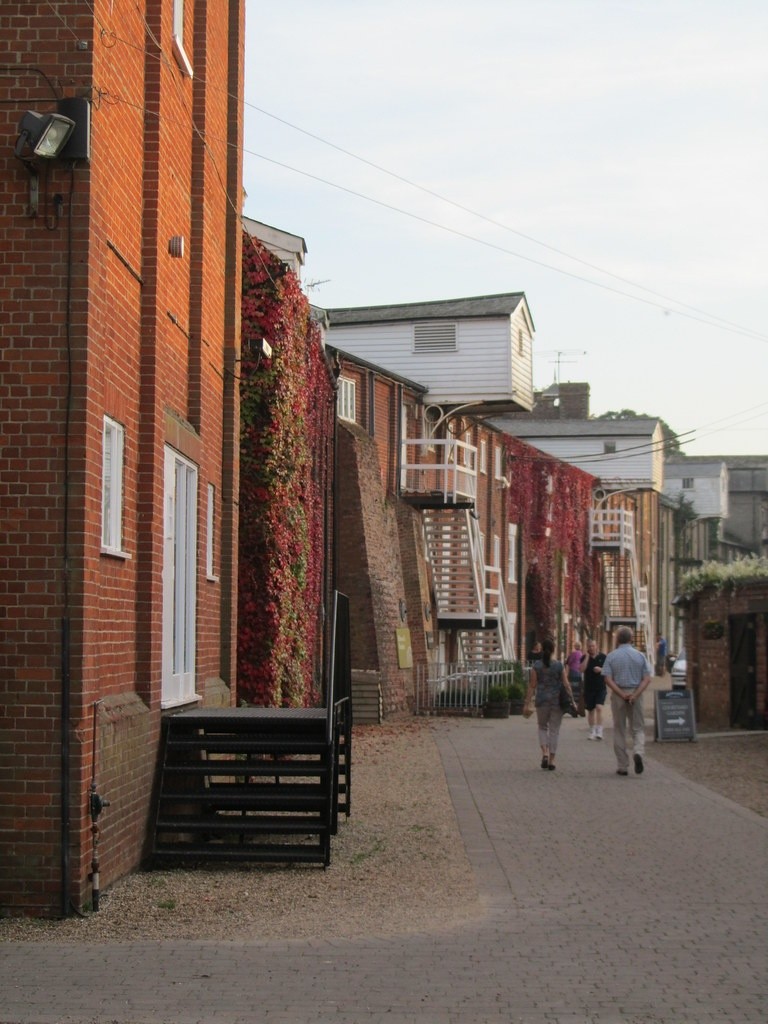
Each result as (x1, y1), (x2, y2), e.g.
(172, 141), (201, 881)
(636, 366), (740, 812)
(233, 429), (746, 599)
(581, 642), (607, 740)
(656, 633), (667, 676)
(522, 638), (577, 770)
(527, 641), (541, 660)
(600, 627), (651, 775)
(564, 642), (585, 717)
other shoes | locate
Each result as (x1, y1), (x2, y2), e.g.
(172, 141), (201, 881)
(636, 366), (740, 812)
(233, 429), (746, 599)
(588, 731), (603, 743)
(541, 756), (548, 769)
(548, 764), (556, 770)
(633, 753), (644, 774)
(617, 768), (628, 775)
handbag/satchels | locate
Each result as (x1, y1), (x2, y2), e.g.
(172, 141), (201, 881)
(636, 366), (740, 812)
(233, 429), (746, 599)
(558, 685), (576, 717)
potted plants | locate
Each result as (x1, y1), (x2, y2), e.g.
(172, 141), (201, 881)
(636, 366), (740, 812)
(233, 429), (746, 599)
(508, 683), (525, 715)
(482, 686), (512, 718)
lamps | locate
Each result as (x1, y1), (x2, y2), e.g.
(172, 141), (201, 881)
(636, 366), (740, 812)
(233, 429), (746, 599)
(14, 110), (75, 219)
(671, 595), (690, 611)
(222, 339), (273, 381)
